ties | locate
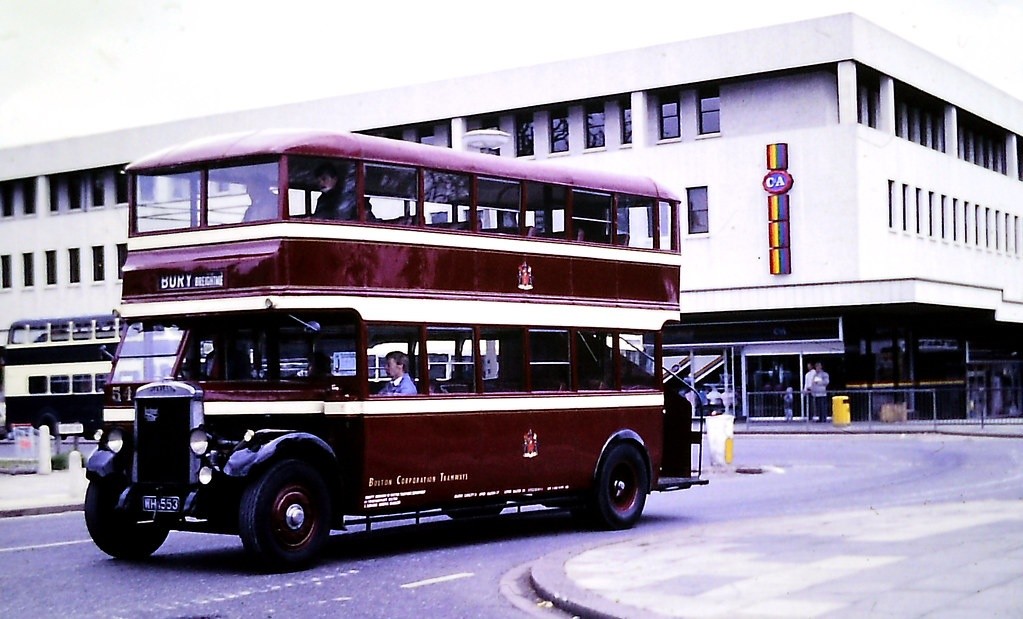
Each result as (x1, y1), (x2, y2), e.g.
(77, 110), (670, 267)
(208, 358), (225, 380)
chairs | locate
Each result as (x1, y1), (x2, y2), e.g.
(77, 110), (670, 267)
(350, 198), (632, 246)
(369, 379), (608, 391)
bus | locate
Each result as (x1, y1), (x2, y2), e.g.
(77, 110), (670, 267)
(83, 122), (709, 575)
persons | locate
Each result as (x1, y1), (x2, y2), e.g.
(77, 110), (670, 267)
(764, 360), (830, 423)
(181, 330), (334, 381)
(378, 352), (418, 396)
(242, 175), (282, 221)
(311, 164), (357, 221)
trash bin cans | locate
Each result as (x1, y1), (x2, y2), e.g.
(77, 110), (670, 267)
(832, 396), (850, 426)
